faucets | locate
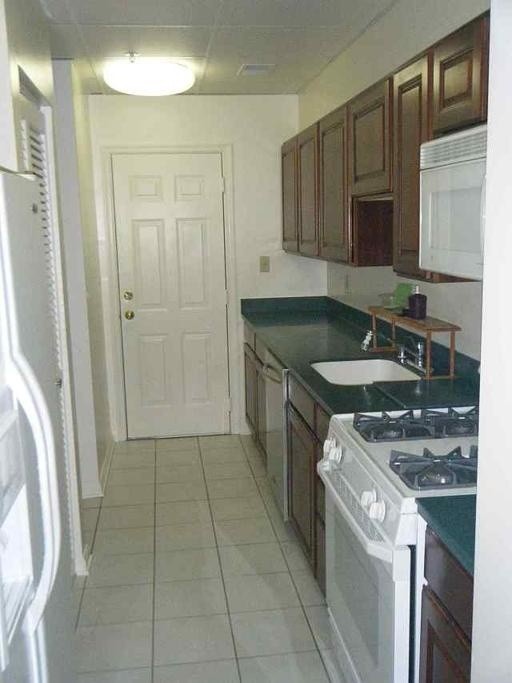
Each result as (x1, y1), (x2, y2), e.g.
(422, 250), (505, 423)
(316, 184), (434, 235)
(360, 328), (407, 364)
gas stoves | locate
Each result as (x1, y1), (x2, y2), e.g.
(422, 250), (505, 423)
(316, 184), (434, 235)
(324, 404), (477, 548)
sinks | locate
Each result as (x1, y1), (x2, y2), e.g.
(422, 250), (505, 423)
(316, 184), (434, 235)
(310, 357), (421, 386)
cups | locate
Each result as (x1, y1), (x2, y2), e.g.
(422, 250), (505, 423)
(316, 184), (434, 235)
(408, 294), (427, 319)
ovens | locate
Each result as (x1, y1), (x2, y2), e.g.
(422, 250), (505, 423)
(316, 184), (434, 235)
(315, 458), (412, 683)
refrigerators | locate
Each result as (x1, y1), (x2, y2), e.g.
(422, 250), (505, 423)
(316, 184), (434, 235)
(1, 168), (72, 683)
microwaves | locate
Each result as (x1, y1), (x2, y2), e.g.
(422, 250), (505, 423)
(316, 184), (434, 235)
(417, 157), (486, 281)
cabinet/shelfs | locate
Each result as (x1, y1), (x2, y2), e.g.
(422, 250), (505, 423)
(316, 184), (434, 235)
(318, 103), (346, 265)
(238, 320), (267, 461)
(280, 120), (319, 259)
(346, 77), (392, 199)
(283, 372), (331, 602)
(430, 10), (488, 135)
(390, 52), (454, 282)
(414, 525), (472, 683)
